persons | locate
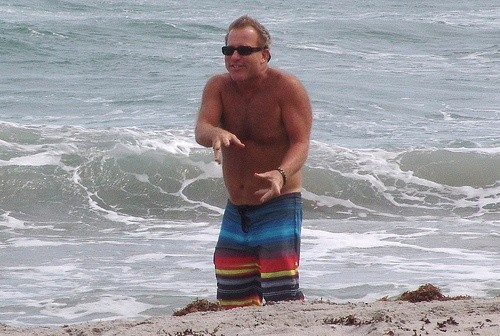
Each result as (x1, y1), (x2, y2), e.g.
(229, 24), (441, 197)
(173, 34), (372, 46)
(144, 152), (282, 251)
(195, 14), (312, 311)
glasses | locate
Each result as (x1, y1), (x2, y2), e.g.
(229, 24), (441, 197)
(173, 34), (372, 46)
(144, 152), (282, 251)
(222, 45), (263, 56)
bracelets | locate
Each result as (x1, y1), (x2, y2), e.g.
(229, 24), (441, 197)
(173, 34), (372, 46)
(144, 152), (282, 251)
(275, 167), (289, 183)
